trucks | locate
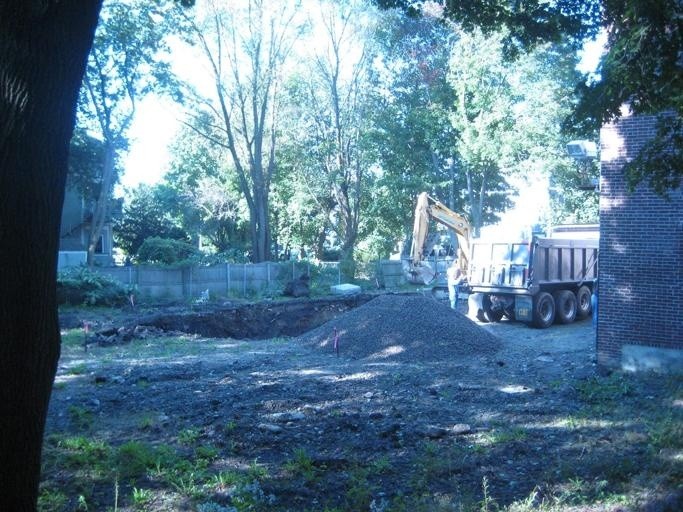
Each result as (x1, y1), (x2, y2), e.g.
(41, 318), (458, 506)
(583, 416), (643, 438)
(467, 224), (599, 328)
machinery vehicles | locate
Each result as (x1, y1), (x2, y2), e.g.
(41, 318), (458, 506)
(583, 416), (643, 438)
(402, 192), (474, 308)
